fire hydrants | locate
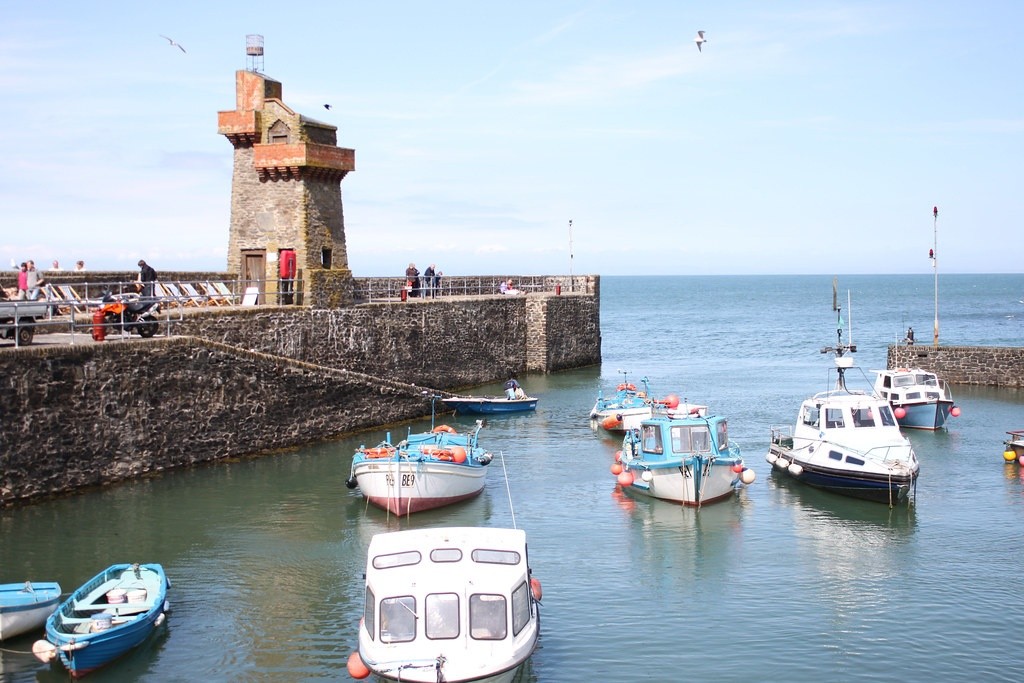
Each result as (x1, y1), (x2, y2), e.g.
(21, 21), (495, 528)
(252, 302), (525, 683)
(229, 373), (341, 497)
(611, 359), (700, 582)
(92, 308), (105, 340)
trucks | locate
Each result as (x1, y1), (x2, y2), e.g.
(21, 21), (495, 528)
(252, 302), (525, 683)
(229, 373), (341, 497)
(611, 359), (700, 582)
(0, 300), (49, 346)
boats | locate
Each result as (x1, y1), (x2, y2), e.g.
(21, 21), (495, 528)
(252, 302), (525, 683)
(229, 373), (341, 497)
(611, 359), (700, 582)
(766, 276), (921, 500)
(357, 450), (540, 683)
(45, 563), (167, 677)
(442, 395), (539, 415)
(0, 581), (62, 642)
(615, 401), (742, 505)
(590, 369), (706, 432)
(1003, 431), (1024, 461)
(351, 426), (487, 517)
(868, 333), (953, 430)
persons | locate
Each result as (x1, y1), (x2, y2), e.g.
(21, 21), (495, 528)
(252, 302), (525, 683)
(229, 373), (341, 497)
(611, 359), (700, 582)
(26, 260), (44, 299)
(139, 260), (157, 296)
(75, 260), (86, 270)
(50, 261), (62, 271)
(500, 279), (528, 296)
(17, 263), (27, 300)
(405, 263), (442, 297)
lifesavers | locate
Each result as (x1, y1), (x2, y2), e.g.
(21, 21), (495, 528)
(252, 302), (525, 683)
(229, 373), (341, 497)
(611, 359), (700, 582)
(362, 447), (396, 458)
(616, 382), (636, 392)
(433, 425), (457, 435)
(420, 448), (453, 462)
(668, 409), (700, 419)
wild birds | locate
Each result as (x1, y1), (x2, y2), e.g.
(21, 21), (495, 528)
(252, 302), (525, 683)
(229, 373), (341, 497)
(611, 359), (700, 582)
(160, 33), (187, 54)
(696, 30), (707, 52)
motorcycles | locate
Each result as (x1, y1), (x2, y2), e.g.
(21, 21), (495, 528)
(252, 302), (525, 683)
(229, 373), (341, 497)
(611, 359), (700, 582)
(99, 288), (162, 338)
